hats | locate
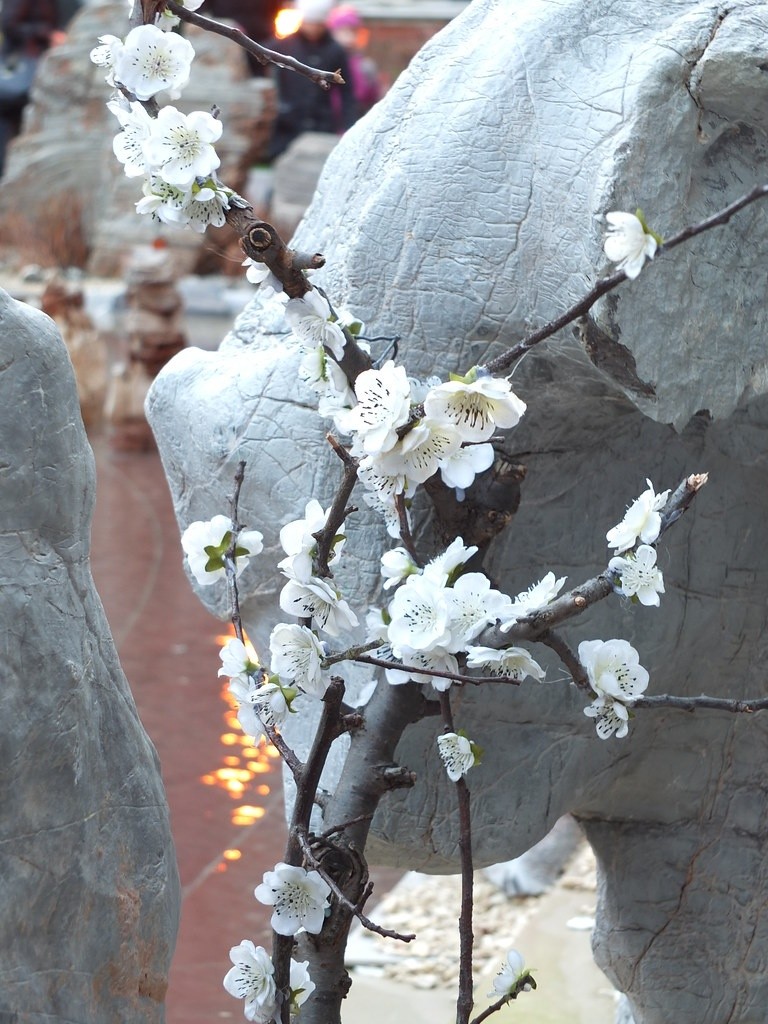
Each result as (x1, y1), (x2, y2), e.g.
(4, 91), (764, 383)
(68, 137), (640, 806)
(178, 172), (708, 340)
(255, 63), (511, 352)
(324, 4), (360, 30)
(299, 0), (330, 24)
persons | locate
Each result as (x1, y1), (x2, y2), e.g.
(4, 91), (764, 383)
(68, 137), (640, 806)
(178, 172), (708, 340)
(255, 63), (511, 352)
(234, 3), (357, 165)
(0, 17), (67, 106)
